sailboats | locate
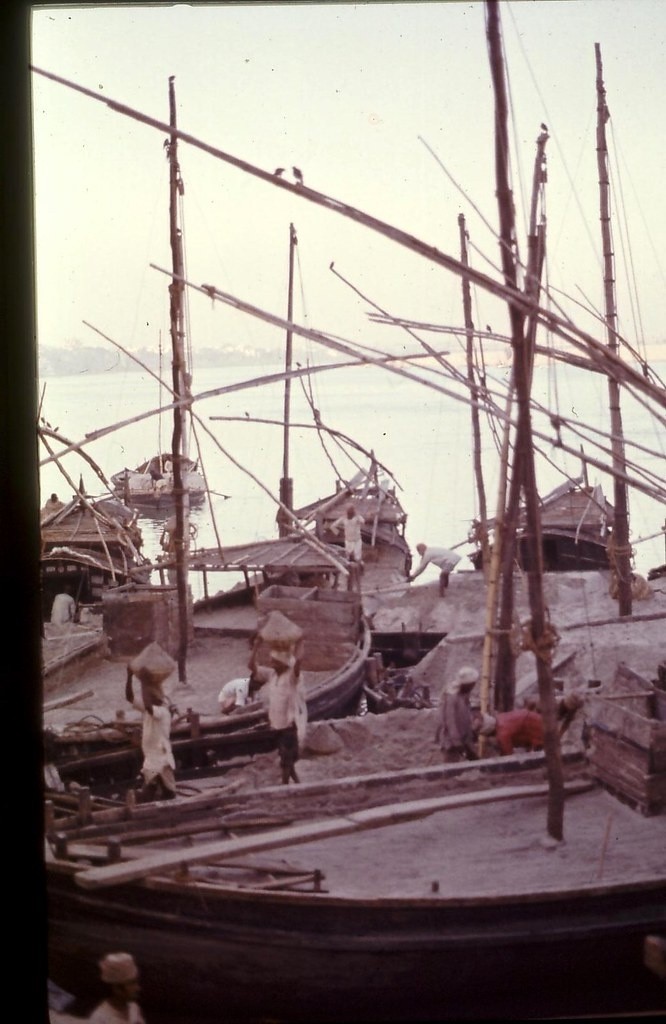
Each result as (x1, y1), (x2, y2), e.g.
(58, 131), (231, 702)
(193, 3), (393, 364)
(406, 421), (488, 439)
(41, 1), (666, 1024)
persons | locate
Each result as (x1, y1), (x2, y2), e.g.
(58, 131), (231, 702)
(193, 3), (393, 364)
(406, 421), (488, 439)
(51, 584), (76, 624)
(88, 952), (145, 1024)
(329, 505), (365, 576)
(247, 632), (304, 785)
(218, 672), (266, 715)
(45, 494), (67, 508)
(434, 666), (480, 776)
(523, 689), (594, 745)
(405, 543), (461, 597)
(478, 709), (545, 757)
(126, 662), (175, 804)
(150, 469), (167, 501)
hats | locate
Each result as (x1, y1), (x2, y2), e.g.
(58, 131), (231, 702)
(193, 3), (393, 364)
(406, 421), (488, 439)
(451, 667), (480, 688)
(99, 950), (137, 982)
(270, 649), (297, 669)
